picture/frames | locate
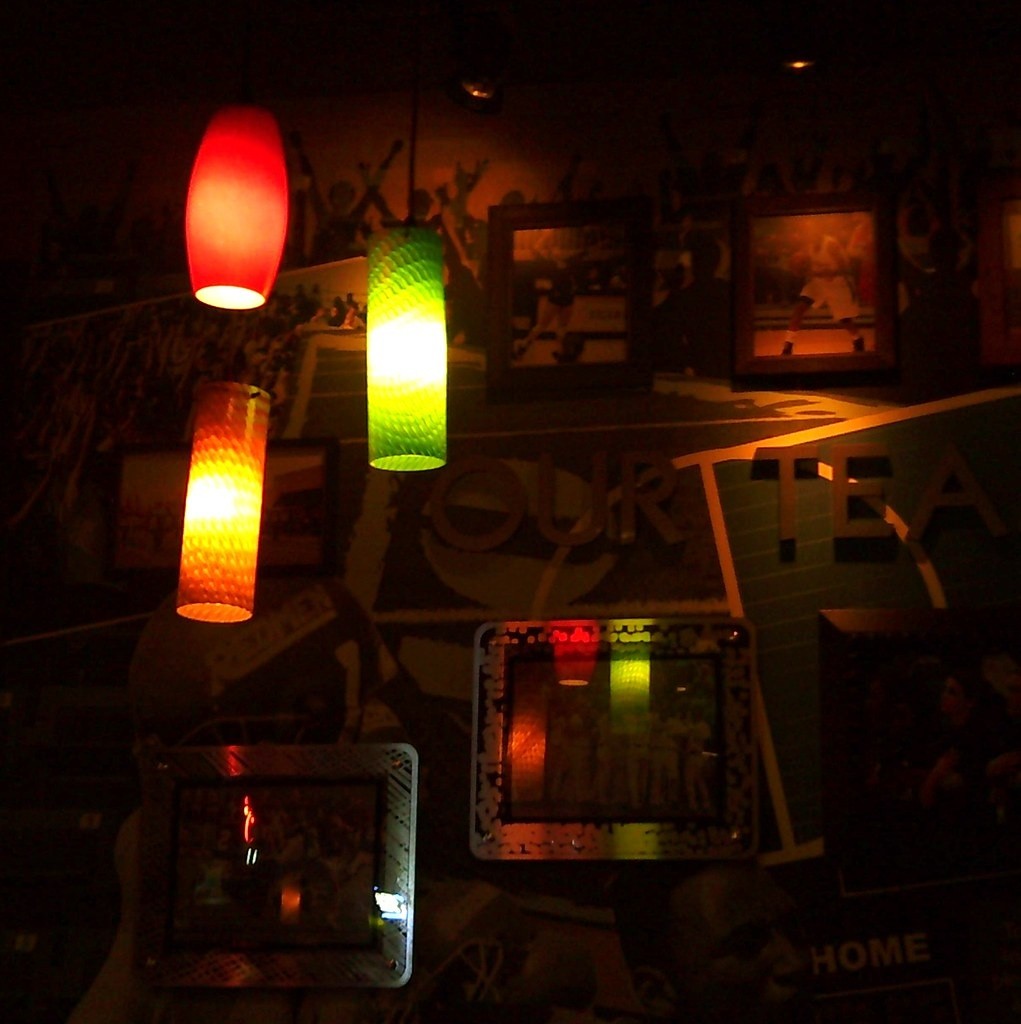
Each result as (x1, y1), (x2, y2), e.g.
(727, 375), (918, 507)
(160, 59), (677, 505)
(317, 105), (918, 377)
(733, 192), (895, 374)
(977, 177), (1021, 370)
(485, 195), (655, 401)
(137, 742), (420, 986)
(470, 616), (758, 861)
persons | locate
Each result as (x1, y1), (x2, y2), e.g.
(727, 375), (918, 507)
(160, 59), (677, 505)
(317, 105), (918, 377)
(547, 693), (712, 813)
(176, 787), (377, 931)
(919, 633), (1021, 843)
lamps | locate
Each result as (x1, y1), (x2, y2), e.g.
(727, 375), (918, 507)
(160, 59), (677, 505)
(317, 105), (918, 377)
(174, 314), (270, 624)
(186, 0), (288, 309)
(367, 0), (448, 470)
